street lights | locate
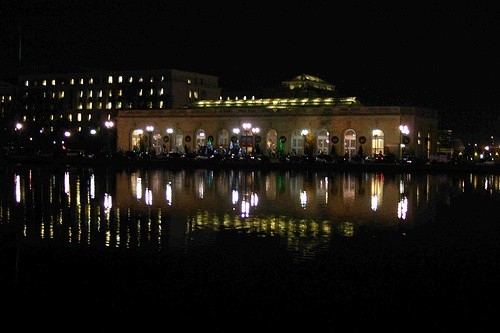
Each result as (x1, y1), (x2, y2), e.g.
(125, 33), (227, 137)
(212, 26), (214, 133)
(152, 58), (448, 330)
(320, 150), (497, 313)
(12, 122), (408, 163)
